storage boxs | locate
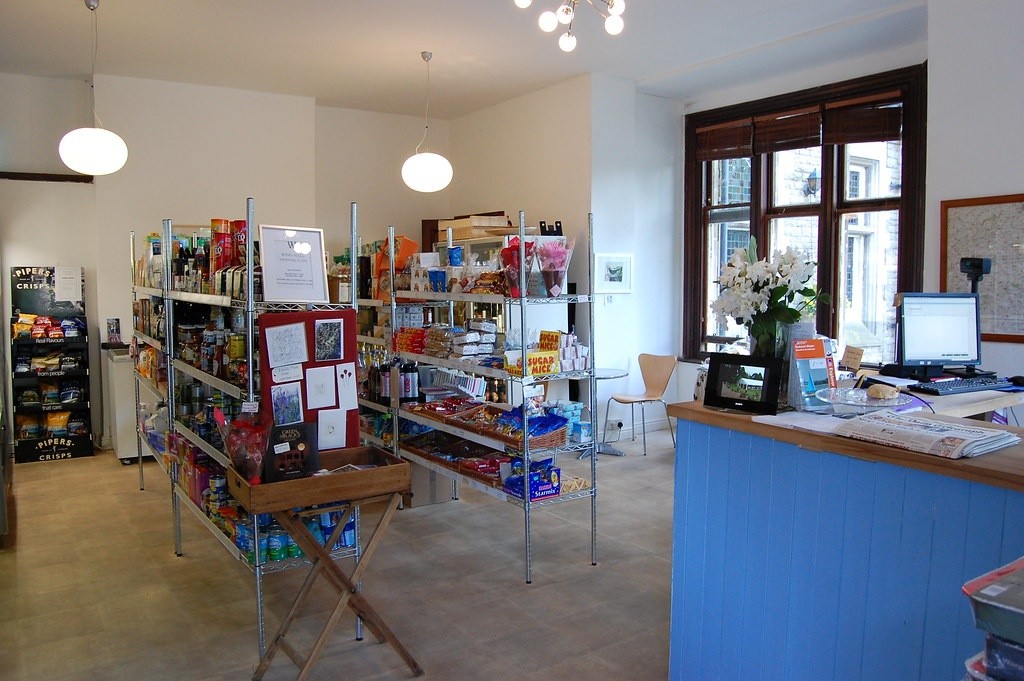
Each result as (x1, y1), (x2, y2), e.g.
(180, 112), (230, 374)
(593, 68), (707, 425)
(227, 446), (412, 515)
(357, 216), (591, 499)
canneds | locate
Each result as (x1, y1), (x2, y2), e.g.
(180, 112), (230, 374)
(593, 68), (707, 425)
(207, 475), (356, 566)
(176, 323), (243, 447)
(207, 218), (246, 296)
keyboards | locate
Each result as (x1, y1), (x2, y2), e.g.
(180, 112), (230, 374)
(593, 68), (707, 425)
(907, 378), (1013, 399)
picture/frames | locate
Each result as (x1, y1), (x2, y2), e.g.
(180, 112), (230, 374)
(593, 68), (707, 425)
(592, 251), (636, 294)
(704, 352), (783, 415)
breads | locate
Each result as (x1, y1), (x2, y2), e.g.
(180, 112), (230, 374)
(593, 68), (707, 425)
(866, 384), (900, 399)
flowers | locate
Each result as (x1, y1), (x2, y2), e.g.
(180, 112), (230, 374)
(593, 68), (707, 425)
(710, 237), (830, 356)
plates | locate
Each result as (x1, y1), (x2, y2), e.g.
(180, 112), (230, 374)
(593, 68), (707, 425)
(486, 227), (536, 234)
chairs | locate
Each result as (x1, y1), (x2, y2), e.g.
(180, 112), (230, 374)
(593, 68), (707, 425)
(603, 353), (678, 455)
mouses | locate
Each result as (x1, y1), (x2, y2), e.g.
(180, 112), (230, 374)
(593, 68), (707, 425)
(1008, 375), (1024, 386)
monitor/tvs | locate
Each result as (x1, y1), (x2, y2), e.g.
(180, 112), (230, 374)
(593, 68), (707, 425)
(899, 292), (982, 381)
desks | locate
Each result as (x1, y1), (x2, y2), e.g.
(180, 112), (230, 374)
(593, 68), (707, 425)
(568, 367), (628, 459)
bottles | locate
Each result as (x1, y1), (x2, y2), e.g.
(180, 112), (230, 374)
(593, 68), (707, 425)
(355, 341), (420, 408)
(199, 306), (245, 381)
(172, 232), (210, 293)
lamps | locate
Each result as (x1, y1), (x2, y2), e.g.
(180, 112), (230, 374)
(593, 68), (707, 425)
(401, 52), (454, 194)
(514, 0), (625, 52)
(803, 167), (821, 197)
(57, 1), (129, 175)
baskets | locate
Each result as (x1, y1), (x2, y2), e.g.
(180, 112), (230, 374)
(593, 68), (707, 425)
(399, 396), (566, 488)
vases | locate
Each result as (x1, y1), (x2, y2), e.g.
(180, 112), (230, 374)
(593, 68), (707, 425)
(751, 322), (792, 412)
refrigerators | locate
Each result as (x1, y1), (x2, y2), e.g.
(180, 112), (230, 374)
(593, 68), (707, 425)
(107, 349), (158, 458)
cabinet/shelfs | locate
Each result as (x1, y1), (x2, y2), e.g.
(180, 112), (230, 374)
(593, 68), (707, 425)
(10, 317), (95, 464)
(130, 198), (363, 663)
(388, 208), (600, 585)
